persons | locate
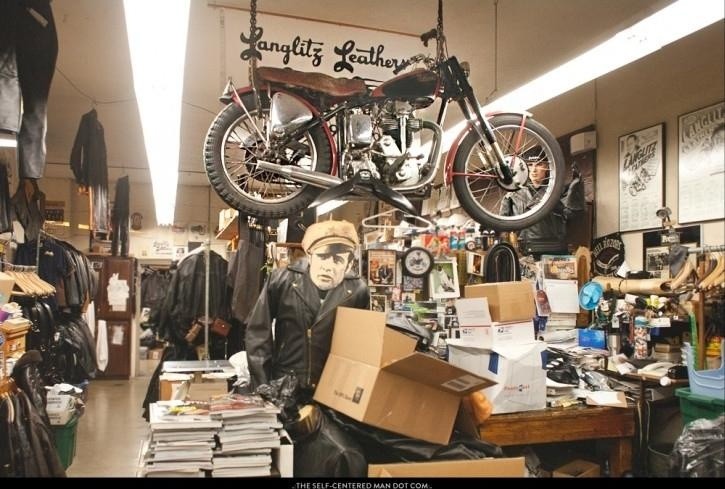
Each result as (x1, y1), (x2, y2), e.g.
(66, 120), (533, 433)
(497, 150), (586, 259)
(622, 135), (641, 159)
(245, 220), (371, 401)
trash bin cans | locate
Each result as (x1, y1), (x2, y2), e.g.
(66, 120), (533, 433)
(51, 415), (77, 469)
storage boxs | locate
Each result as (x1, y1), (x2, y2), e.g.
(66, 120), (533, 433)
(675, 387), (724, 427)
(552, 459), (599, 478)
(50, 412), (79, 470)
(311, 306), (500, 448)
(464, 280), (540, 323)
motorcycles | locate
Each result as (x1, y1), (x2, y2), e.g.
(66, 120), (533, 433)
(202, 29), (566, 232)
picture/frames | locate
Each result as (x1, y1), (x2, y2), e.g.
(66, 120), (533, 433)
(618, 123), (666, 233)
(677, 99), (724, 225)
(402, 247), (433, 277)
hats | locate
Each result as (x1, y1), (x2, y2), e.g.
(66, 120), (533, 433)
(523, 145), (549, 164)
(302, 220), (360, 256)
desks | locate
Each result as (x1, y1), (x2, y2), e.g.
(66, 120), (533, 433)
(478, 401), (640, 478)
(598, 368), (689, 477)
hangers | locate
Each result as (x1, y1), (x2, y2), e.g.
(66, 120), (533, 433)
(4, 261), (57, 301)
(668, 244), (725, 293)
(362, 208), (433, 231)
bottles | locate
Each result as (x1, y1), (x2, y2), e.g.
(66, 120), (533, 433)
(450, 231), (466, 250)
(634, 317), (648, 360)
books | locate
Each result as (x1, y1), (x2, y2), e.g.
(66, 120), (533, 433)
(136, 395), (284, 477)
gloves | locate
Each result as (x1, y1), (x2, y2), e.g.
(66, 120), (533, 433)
(563, 161), (584, 211)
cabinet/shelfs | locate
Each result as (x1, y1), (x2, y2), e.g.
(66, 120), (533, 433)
(87, 254), (136, 379)
